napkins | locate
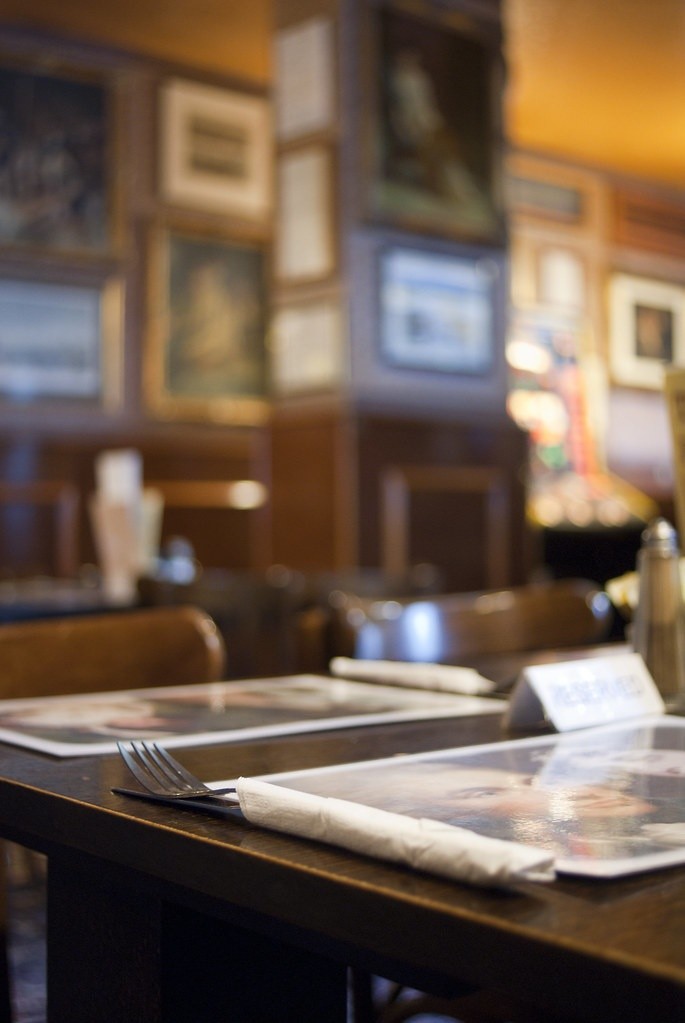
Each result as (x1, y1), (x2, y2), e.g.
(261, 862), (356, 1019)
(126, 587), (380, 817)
(234, 775), (556, 885)
(330, 657), (500, 697)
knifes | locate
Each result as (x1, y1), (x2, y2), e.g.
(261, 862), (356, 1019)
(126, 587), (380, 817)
(110, 788), (247, 825)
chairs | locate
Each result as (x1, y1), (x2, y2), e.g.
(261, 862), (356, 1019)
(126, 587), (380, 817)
(1, 606), (226, 701)
(326, 573), (614, 663)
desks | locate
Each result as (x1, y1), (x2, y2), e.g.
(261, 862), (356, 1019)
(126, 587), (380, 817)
(1, 670), (685, 1023)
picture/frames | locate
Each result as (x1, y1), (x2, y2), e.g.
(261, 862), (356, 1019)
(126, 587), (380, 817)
(1, 1), (685, 594)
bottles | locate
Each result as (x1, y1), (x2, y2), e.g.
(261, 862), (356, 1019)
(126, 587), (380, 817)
(637, 520), (685, 713)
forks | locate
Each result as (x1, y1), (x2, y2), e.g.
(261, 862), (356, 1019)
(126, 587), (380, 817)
(117, 741), (238, 803)
